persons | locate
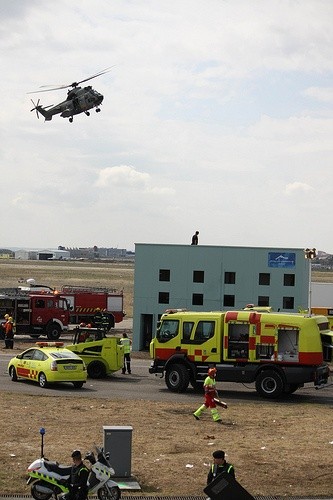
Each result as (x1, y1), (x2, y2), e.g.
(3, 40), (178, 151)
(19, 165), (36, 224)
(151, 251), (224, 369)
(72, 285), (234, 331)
(2, 314), (14, 349)
(57, 450), (89, 500)
(78, 322), (92, 343)
(120, 333), (132, 374)
(207, 450), (236, 485)
(192, 368), (222, 422)
(191, 231), (199, 245)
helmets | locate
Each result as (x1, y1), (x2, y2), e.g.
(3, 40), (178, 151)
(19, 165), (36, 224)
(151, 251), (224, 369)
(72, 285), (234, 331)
(8, 317), (12, 321)
(4, 314), (9, 318)
(208, 368), (216, 376)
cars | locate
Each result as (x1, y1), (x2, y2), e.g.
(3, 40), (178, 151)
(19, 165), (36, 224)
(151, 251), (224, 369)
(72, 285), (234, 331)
(7, 342), (87, 387)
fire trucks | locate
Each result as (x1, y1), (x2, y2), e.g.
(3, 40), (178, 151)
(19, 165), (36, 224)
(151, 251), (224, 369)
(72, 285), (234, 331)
(18, 285), (125, 331)
(148, 304), (333, 400)
(0, 278), (72, 341)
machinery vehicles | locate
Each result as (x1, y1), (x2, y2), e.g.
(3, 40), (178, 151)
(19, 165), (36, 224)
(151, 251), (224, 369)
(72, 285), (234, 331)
(56, 323), (125, 379)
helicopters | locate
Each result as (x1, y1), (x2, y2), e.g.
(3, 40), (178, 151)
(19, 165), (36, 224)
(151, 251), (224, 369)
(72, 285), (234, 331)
(24, 68), (110, 123)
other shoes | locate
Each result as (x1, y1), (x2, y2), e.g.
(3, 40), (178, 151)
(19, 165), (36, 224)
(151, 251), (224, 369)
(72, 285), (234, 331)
(214, 419), (222, 422)
(129, 372), (131, 374)
(3, 347), (13, 349)
(121, 372), (125, 374)
(193, 414), (200, 420)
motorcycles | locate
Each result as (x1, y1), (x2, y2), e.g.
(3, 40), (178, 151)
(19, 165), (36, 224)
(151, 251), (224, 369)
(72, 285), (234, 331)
(25, 427), (121, 500)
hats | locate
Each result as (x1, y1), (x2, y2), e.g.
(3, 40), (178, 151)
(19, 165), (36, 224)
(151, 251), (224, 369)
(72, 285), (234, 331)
(213, 451), (225, 458)
(71, 451), (81, 458)
